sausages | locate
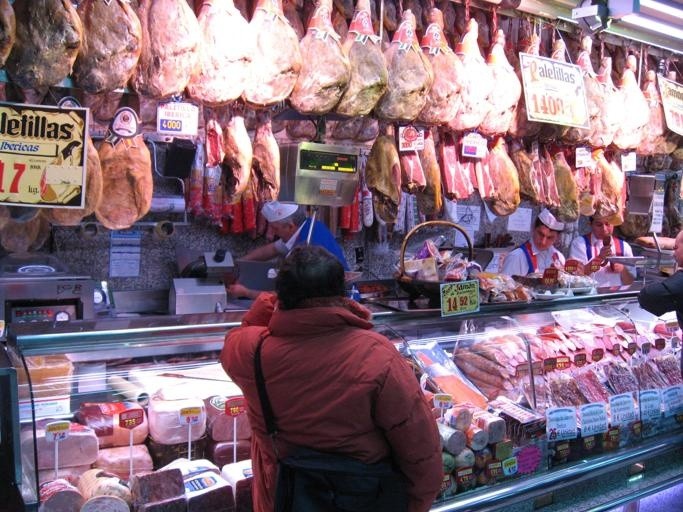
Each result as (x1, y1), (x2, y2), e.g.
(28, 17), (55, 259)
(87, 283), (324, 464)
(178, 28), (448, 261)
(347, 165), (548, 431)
(453, 320), (683, 399)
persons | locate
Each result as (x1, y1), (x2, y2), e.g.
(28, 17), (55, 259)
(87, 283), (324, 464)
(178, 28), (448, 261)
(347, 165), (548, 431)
(568, 216), (637, 285)
(221, 244), (443, 512)
(502, 208), (566, 277)
(226, 200), (360, 303)
(638, 230), (683, 378)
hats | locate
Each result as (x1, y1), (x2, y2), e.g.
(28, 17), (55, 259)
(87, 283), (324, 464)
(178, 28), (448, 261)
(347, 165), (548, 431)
(538, 208), (565, 231)
(260, 201), (299, 223)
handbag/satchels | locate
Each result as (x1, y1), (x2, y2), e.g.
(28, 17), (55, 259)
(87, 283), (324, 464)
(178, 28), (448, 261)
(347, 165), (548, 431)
(274, 444), (407, 512)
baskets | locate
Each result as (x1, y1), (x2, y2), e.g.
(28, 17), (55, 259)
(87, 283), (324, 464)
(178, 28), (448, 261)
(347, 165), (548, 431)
(394, 220), (473, 299)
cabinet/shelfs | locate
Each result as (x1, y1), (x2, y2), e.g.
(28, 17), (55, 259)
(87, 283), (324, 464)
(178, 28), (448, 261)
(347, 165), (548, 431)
(11, 289), (683, 512)
